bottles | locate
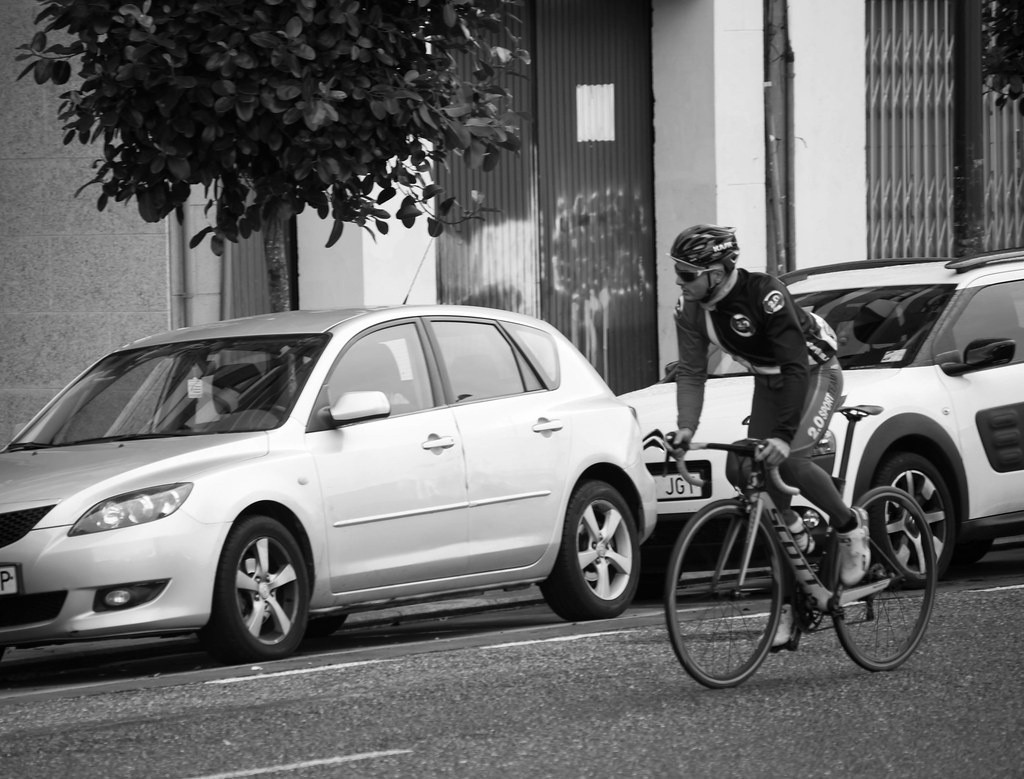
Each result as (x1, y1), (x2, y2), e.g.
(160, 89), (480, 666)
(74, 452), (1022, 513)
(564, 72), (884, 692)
(782, 506), (815, 553)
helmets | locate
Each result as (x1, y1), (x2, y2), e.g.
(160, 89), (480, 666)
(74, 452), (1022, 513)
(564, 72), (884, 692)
(670, 224), (739, 277)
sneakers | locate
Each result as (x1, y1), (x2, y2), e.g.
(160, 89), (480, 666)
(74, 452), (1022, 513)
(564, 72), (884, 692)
(757, 604), (794, 646)
(836, 506), (871, 585)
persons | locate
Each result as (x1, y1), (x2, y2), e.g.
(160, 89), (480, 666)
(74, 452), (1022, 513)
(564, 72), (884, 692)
(663, 223), (871, 647)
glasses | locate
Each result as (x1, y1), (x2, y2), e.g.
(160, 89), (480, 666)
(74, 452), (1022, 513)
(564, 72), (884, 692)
(675, 264), (720, 282)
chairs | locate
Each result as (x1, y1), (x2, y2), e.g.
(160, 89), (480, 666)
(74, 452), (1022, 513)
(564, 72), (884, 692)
(330, 344), (401, 399)
(854, 296), (901, 352)
(212, 364), (262, 416)
